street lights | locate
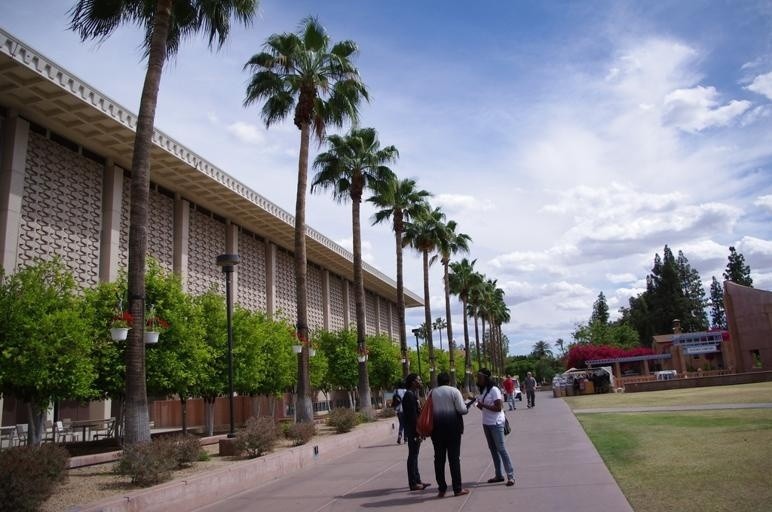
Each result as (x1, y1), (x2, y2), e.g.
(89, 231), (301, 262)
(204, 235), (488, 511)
(214, 252), (245, 439)
(410, 328), (423, 383)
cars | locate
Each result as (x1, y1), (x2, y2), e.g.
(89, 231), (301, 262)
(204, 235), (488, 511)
(503, 377), (522, 403)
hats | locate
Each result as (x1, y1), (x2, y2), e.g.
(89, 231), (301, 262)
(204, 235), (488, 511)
(477, 369), (491, 376)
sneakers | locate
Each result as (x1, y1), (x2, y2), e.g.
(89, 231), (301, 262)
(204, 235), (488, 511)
(411, 477), (515, 496)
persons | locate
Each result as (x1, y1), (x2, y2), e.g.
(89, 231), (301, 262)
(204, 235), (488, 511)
(393, 383), (410, 443)
(425, 372), (470, 498)
(503, 375), (517, 410)
(523, 371), (536, 409)
(468, 368), (515, 487)
(401, 373), (431, 491)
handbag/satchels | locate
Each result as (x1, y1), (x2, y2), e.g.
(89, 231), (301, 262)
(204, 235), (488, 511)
(392, 395), (402, 407)
(416, 395), (434, 437)
(504, 418), (511, 435)
